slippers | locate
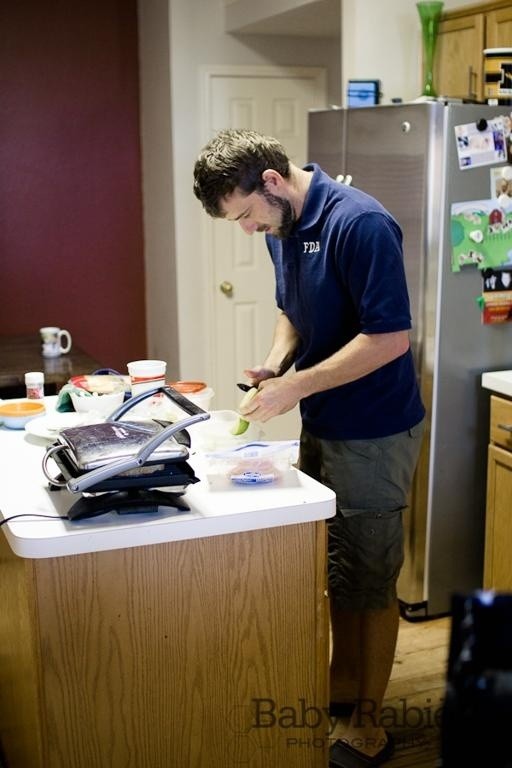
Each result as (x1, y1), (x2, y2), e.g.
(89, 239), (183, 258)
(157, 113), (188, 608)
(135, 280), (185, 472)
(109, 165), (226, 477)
(329, 701), (355, 718)
(329, 730), (396, 767)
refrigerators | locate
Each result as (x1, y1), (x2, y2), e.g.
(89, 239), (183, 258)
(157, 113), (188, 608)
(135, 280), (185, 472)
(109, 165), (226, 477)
(301, 96), (512, 622)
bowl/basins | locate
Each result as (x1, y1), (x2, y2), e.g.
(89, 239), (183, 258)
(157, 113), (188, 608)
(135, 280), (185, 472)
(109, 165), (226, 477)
(68, 391), (126, 414)
(1, 402), (47, 430)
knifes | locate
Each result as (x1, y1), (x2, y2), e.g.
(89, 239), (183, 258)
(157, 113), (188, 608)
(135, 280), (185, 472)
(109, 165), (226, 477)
(236, 383), (254, 393)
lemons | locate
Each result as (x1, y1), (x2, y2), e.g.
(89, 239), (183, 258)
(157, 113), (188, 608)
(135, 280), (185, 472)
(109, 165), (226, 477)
(228, 418), (249, 435)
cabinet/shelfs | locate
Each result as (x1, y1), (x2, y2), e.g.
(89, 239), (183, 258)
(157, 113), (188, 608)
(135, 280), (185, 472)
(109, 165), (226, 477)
(422, 0), (512, 103)
(483, 391), (512, 593)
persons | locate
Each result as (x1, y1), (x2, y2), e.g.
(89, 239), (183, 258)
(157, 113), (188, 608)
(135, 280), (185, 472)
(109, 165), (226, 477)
(193, 131), (424, 768)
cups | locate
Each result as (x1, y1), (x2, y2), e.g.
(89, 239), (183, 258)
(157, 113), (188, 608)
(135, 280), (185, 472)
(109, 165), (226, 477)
(24, 371), (45, 400)
(126, 358), (167, 398)
(39, 326), (73, 359)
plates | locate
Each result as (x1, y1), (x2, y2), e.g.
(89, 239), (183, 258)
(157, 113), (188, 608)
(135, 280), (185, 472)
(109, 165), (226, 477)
(25, 412), (106, 440)
